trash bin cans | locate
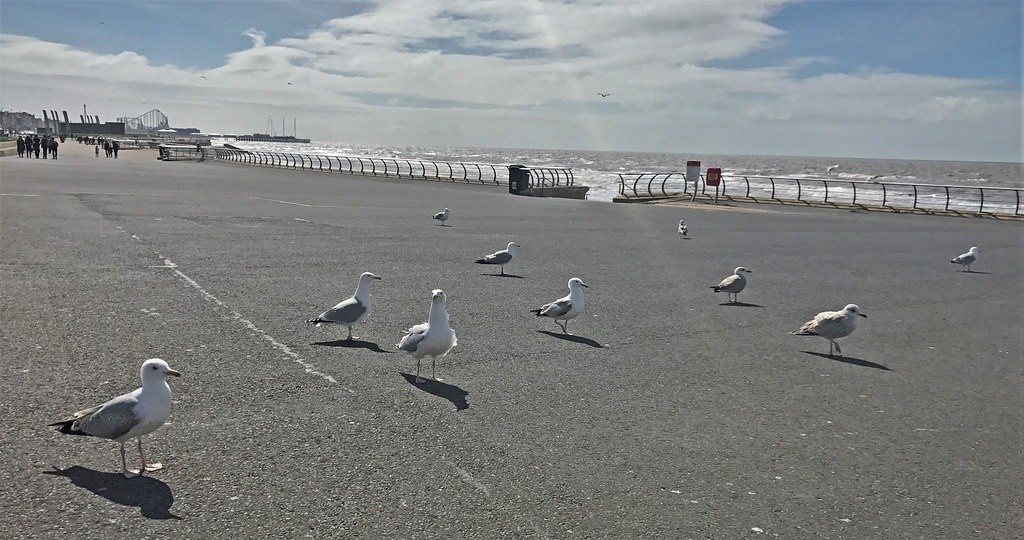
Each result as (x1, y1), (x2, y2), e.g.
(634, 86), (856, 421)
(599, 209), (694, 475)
(508, 164), (530, 195)
(159, 144), (170, 156)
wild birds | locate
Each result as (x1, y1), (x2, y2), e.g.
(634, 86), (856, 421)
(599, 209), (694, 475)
(432, 208), (452, 226)
(529, 277), (590, 334)
(477, 240), (520, 278)
(595, 92), (612, 97)
(790, 303), (867, 356)
(304, 272), (384, 339)
(679, 219), (688, 239)
(288, 82), (293, 86)
(394, 291), (459, 384)
(947, 247), (984, 270)
(200, 75), (207, 80)
(52, 357), (183, 474)
(709, 266), (753, 304)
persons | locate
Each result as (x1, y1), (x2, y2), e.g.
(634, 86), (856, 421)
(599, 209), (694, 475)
(95, 146), (99, 157)
(104, 141), (119, 158)
(17, 134), (58, 159)
(161, 135), (175, 140)
(71, 133), (106, 146)
